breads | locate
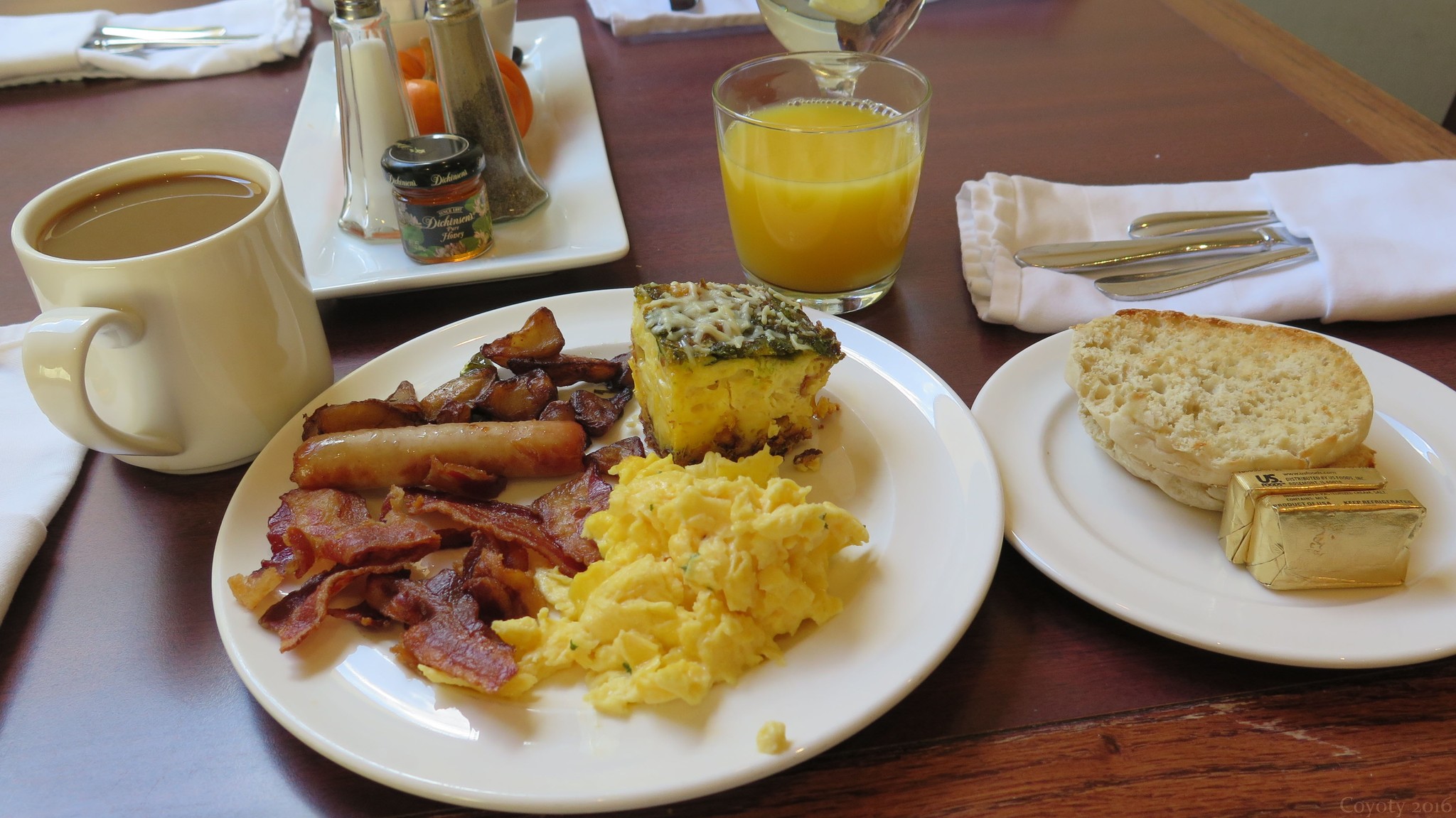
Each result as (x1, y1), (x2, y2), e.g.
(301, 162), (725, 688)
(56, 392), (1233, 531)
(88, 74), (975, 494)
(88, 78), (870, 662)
(1067, 308), (1379, 511)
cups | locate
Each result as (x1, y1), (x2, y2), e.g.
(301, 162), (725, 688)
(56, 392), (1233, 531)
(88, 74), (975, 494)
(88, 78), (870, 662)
(706, 47), (930, 316)
(13, 139), (334, 479)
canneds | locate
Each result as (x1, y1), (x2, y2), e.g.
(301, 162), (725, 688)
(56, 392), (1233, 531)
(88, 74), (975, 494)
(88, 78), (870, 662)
(382, 133), (495, 265)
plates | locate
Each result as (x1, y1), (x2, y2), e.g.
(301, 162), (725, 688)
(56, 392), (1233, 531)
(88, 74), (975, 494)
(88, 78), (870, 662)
(275, 18), (630, 301)
(213, 287), (1007, 815)
(961, 308), (1456, 674)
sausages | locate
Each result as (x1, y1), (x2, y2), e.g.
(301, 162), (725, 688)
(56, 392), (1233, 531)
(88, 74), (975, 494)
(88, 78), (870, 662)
(290, 418), (585, 489)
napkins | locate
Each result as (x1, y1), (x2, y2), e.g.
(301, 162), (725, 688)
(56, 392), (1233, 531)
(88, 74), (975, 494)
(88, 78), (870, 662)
(952, 157), (1456, 335)
(0, 0), (312, 96)
(0, 325), (88, 630)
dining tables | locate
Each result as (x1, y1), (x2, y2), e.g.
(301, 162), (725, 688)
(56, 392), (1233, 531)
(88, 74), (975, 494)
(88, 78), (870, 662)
(1, 0), (1456, 818)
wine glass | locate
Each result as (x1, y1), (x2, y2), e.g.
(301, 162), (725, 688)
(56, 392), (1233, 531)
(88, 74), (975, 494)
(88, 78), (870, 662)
(753, 1), (926, 103)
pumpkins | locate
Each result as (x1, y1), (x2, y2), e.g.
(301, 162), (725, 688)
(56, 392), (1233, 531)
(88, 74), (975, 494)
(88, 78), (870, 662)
(396, 38), (534, 137)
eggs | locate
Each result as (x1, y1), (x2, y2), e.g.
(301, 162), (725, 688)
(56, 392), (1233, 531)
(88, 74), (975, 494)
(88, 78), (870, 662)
(415, 446), (880, 722)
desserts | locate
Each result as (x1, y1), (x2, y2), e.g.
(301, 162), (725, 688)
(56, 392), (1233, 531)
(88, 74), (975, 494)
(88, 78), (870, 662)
(626, 280), (847, 466)
